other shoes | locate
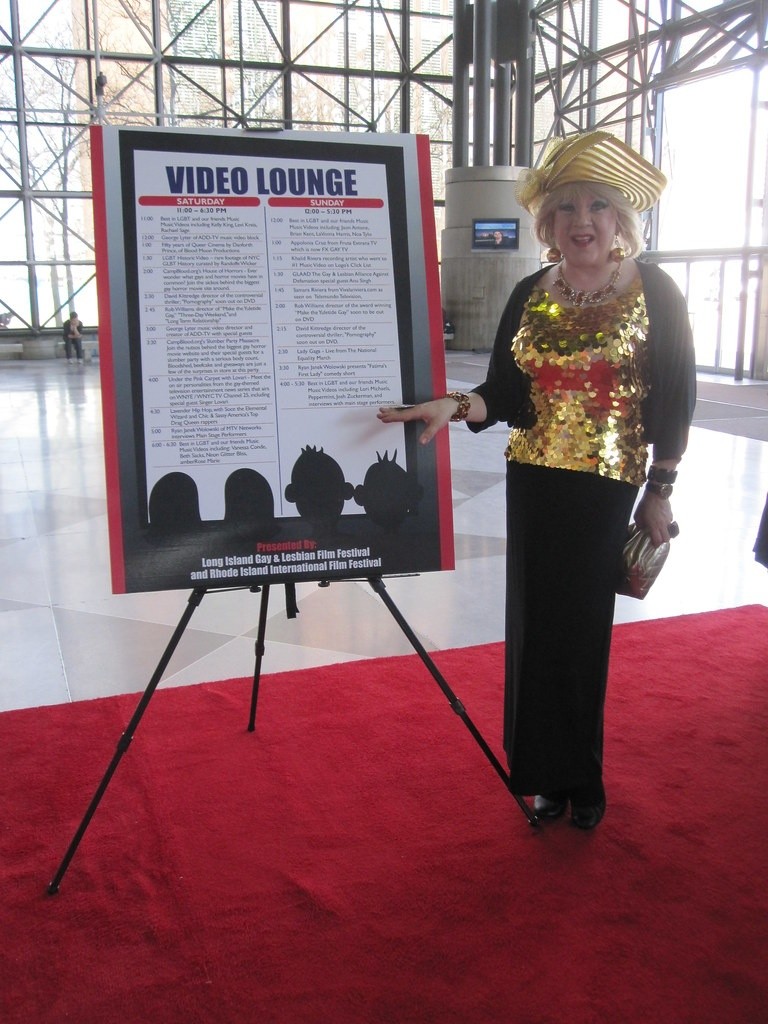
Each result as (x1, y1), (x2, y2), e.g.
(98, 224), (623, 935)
(66, 358), (73, 364)
(77, 358), (83, 364)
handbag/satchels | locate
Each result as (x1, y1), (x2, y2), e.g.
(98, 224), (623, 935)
(620, 523), (678, 599)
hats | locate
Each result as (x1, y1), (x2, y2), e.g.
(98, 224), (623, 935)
(515, 131), (666, 219)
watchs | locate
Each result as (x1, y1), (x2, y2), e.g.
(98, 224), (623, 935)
(646, 485), (675, 499)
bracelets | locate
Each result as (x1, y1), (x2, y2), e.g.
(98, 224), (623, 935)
(442, 391), (471, 422)
(647, 465), (678, 484)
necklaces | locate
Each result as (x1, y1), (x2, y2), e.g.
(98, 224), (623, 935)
(551, 261), (625, 307)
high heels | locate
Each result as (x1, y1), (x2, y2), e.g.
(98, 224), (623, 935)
(529, 779), (609, 831)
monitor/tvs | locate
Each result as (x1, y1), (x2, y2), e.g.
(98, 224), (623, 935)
(471, 218), (520, 252)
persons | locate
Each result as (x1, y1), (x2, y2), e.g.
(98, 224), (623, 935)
(492, 231), (509, 245)
(63, 312), (84, 365)
(0, 313), (14, 329)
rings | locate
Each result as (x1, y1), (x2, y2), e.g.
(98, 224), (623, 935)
(377, 132), (697, 827)
(390, 404), (415, 409)
